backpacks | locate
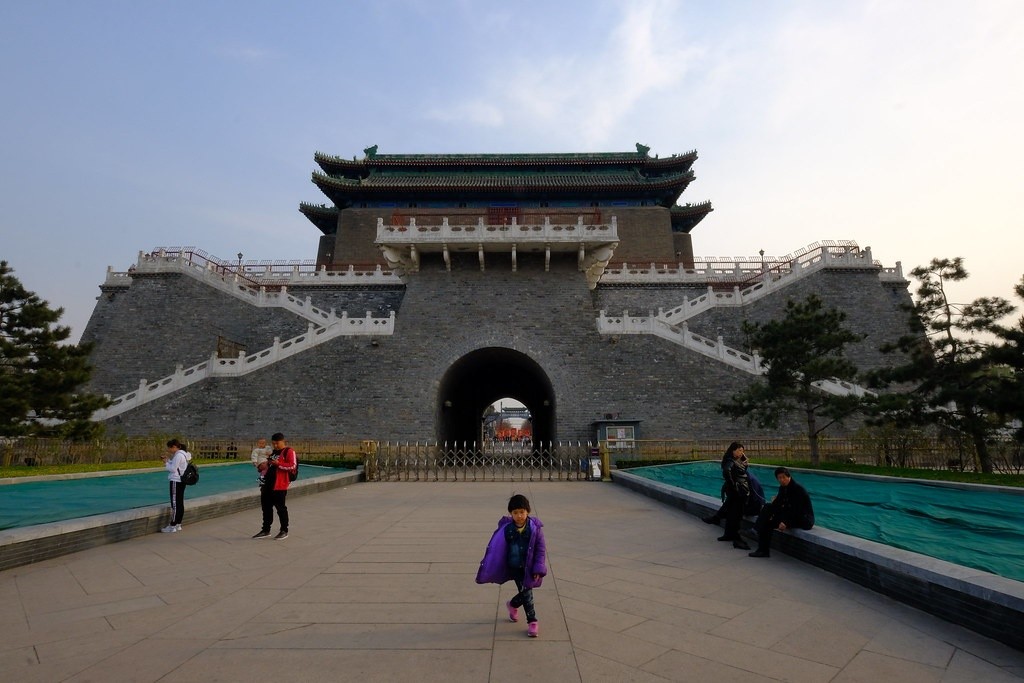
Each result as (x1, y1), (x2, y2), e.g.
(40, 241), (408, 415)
(172, 452), (199, 486)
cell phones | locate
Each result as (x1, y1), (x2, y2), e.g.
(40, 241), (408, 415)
(740, 453), (747, 462)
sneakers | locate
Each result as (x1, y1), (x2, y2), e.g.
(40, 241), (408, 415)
(718, 534), (751, 550)
(252, 531), (271, 539)
(161, 524), (181, 533)
(749, 547), (771, 557)
(274, 530), (289, 540)
(505, 601), (518, 621)
(528, 622), (539, 637)
(702, 515), (721, 525)
(256, 477), (266, 485)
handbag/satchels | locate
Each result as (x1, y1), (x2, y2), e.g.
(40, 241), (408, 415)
(283, 447), (298, 481)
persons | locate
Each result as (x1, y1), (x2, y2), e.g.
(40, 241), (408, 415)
(738, 466), (815, 558)
(250, 431), (298, 540)
(717, 441), (766, 549)
(475, 489), (547, 637)
(160, 438), (191, 534)
